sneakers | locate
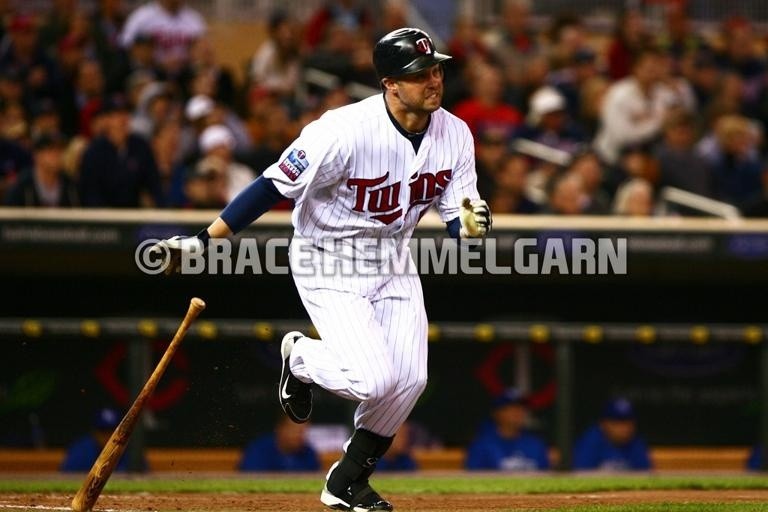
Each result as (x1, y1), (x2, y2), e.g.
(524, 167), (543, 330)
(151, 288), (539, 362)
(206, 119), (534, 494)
(280, 332), (313, 422)
(320, 462), (393, 512)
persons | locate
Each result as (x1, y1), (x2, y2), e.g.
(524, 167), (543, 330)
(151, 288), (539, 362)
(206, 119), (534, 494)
(372, 424), (423, 473)
(572, 398), (655, 471)
(234, 406), (326, 474)
(462, 388), (553, 473)
(0, 2), (768, 225)
(146, 28), (495, 512)
(59, 407), (153, 474)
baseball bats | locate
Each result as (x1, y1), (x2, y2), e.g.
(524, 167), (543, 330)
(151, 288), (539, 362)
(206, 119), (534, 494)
(69, 296), (206, 510)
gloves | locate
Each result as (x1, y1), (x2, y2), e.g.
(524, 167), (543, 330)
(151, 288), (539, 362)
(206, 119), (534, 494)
(149, 228), (213, 274)
(459, 197), (492, 238)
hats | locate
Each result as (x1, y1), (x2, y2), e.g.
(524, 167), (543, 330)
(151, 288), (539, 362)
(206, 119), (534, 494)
(97, 410), (118, 427)
(498, 386), (522, 405)
(605, 397), (632, 417)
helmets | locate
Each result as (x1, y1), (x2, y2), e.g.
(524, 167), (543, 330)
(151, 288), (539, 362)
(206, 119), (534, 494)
(373, 28), (452, 76)
